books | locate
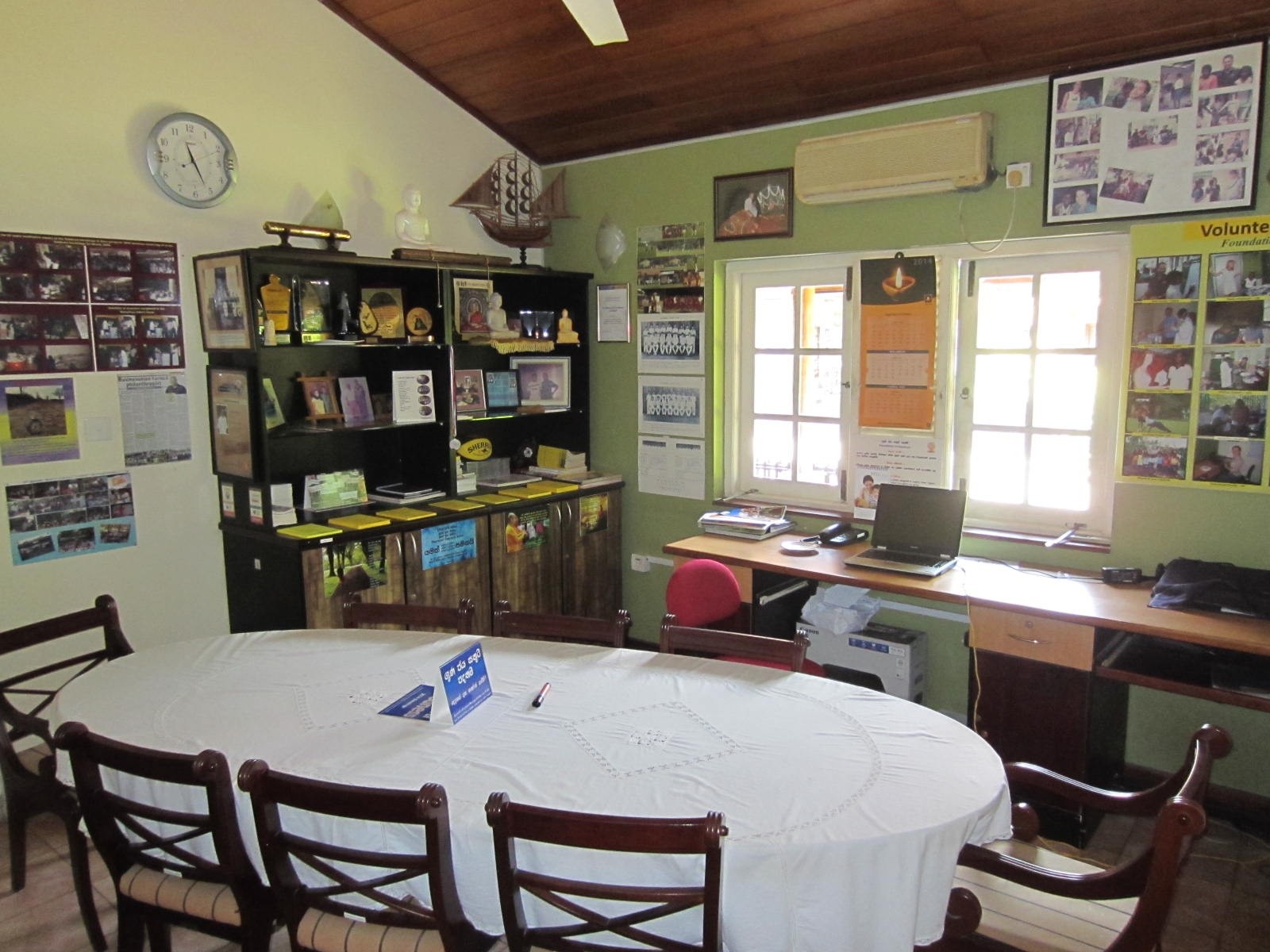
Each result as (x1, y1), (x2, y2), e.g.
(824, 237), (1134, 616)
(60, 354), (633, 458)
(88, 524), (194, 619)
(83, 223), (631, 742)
(456, 443), (622, 489)
(697, 506), (797, 540)
(367, 481), (447, 504)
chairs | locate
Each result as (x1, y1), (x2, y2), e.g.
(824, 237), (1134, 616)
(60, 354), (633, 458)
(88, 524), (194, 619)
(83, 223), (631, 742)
(0, 593), (1235, 952)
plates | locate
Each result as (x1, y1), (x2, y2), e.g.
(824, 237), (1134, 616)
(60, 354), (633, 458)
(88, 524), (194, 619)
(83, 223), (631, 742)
(406, 307), (433, 336)
(781, 541), (819, 551)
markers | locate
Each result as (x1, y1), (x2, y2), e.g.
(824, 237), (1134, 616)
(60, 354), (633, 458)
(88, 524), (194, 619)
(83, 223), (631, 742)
(532, 682), (551, 708)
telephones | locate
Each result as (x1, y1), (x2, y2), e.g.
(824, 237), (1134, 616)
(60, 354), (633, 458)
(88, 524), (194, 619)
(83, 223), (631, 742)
(818, 522), (869, 547)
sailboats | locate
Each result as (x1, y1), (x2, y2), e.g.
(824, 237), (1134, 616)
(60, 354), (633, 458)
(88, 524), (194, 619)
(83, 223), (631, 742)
(448, 152), (579, 249)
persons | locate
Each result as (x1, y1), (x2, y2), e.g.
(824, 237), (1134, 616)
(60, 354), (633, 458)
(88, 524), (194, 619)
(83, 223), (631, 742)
(0, 237), (185, 373)
(505, 512), (528, 553)
(743, 192), (761, 218)
(641, 320), (701, 360)
(1214, 446), (1245, 481)
(641, 386), (700, 428)
(526, 372), (558, 400)
(1207, 399), (1249, 436)
(1119, 249), (1203, 482)
(311, 378), (369, 423)
(462, 292), (521, 338)
(394, 185), (439, 250)
(1208, 250), (1264, 389)
(165, 376), (187, 395)
(1051, 49), (1254, 218)
(558, 308), (574, 334)
(858, 475), (880, 506)
(5, 417), (134, 561)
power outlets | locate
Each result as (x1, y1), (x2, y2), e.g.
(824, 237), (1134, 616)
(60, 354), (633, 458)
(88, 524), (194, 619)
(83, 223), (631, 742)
(1006, 162), (1031, 190)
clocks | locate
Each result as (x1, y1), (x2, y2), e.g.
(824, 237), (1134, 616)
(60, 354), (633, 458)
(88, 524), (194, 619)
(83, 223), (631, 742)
(146, 112), (239, 210)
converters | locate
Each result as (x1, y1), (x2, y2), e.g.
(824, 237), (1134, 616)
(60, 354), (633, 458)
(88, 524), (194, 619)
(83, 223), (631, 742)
(1102, 567), (1142, 583)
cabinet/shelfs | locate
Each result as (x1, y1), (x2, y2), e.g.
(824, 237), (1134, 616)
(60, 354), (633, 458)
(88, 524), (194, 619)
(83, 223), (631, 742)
(218, 248), (626, 637)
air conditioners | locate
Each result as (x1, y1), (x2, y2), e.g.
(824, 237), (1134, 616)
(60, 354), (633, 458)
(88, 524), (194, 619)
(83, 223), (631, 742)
(794, 110), (995, 204)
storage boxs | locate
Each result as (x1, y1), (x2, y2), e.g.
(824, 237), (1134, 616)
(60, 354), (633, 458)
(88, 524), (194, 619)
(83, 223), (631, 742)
(795, 614), (928, 708)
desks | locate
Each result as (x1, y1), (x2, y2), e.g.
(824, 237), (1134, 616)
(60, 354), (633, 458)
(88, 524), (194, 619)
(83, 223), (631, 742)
(47, 628), (1013, 952)
(662, 532), (1270, 853)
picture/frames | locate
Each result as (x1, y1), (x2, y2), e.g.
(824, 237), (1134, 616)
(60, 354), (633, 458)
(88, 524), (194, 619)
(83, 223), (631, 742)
(191, 249), (258, 355)
(713, 166), (793, 241)
(454, 356), (571, 409)
(355, 281), (409, 345)
(1041, 33), (1270, 229)
(262, 370), (344, 434)
(204, 364), (260, 489)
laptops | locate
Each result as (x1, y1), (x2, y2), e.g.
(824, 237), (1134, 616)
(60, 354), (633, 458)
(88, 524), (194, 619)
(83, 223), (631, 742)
(844, 484), (967, 576)
